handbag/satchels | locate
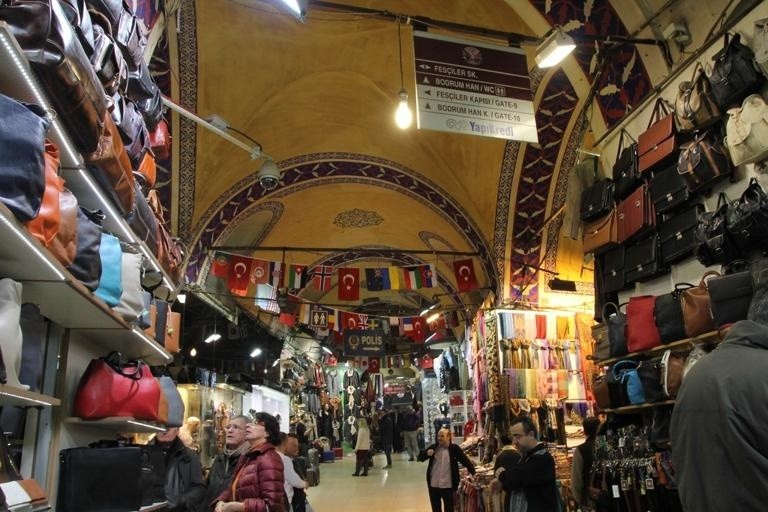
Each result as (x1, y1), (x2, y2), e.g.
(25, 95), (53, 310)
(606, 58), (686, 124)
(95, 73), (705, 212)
(0, 354), (184, 511)
(580, 31), (767, 409)
(0, 0), (188, 350)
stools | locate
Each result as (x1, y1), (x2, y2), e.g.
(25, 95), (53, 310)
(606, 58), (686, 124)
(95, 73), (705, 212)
(332, 448), (343, 458)
(324, 451), (334, 461)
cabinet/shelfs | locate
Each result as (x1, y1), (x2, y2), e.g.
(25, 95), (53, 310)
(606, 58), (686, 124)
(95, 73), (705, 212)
(0, 0), (176, 368)
(447, 390), (473, 440)
(64, 414), (168, 511)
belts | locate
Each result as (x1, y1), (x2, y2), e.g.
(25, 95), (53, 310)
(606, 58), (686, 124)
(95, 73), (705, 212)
(589, 423), (683, 512)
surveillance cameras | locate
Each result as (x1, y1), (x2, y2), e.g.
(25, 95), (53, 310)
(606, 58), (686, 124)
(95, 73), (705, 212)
(257, 159), (280, 191)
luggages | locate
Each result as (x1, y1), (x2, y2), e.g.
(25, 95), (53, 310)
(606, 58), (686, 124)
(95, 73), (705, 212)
(294, 448), (320, 486)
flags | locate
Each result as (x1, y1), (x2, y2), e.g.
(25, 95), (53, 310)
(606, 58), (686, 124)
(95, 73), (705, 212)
(210, 250), (480, 373)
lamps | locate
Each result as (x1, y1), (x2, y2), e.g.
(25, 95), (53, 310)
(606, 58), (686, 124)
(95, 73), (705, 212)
(290, 334), (333, 355)
(548, 270), (576, 292)
(419, 287), (497, 323)
(269, 0), (313, 25)
(533, 23), (575, 69)
(425, 328), (454, 343)
(393, 16), (412, 130)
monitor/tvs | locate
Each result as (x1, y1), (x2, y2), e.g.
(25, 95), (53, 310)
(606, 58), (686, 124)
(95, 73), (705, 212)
(235, 302), (282, 392)
(172, 290), (240, 383)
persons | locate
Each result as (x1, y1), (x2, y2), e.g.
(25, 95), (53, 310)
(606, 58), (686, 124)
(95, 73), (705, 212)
(418, 428), (476, 512)
(141, 411), (317, 512)
(488, 416), (560, 511)
(669, 284), (768, 512)
(351, 405), (422, 476)
(571, 416), (601, 512)
(495, 435), (522, 478)
